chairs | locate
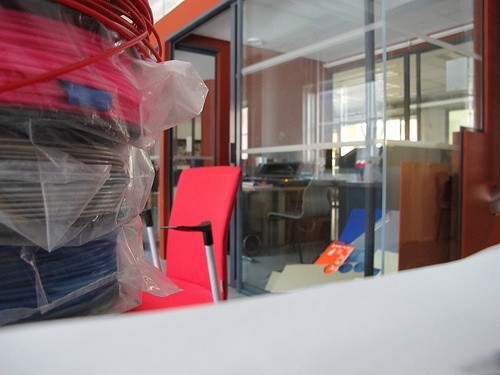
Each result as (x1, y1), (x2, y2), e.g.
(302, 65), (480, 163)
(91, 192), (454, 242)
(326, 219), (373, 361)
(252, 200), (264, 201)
(107, 166), (243, 313)
(266, 179), (347, 266)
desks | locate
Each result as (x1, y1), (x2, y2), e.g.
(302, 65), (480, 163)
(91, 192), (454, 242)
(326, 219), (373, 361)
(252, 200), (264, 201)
(239, 173), (382, 247)
